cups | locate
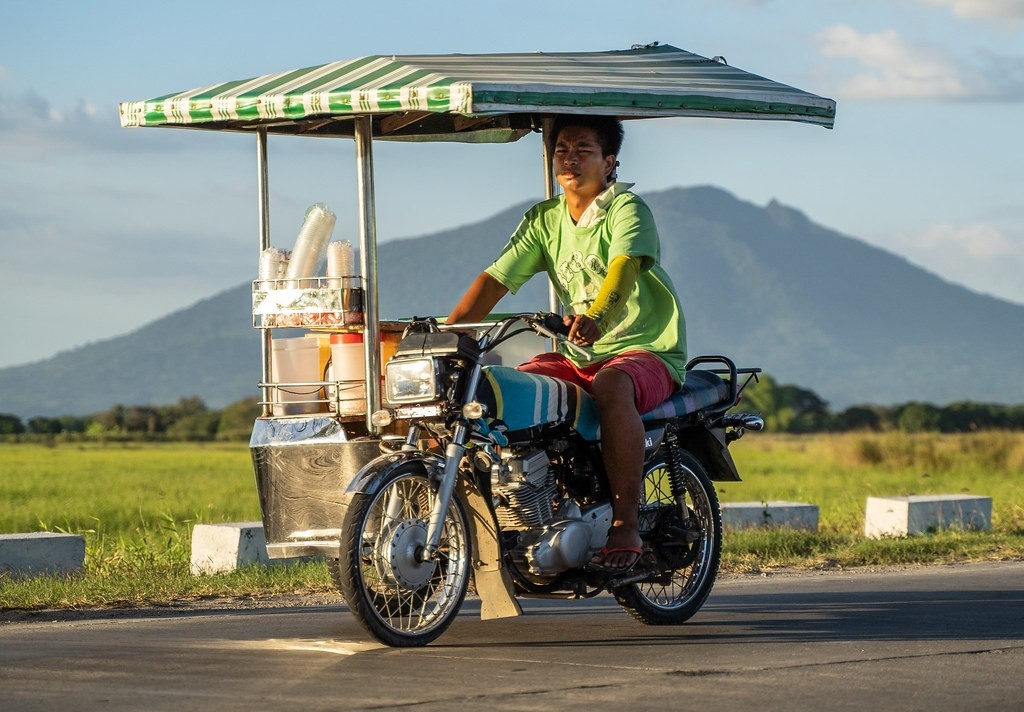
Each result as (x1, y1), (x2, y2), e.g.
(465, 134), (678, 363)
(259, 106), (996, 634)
(258, 205), (355, 289)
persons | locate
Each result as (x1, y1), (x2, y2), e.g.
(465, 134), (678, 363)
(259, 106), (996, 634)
(443, 114), (687, 577)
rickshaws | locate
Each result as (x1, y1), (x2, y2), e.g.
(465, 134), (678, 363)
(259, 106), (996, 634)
(118, 40), (837, 648)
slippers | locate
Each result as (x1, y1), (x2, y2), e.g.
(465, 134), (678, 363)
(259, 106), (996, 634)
(587, 545), (644, 576)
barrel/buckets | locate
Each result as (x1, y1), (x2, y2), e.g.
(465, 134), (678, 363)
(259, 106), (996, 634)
(271, 333), (402, 417)
